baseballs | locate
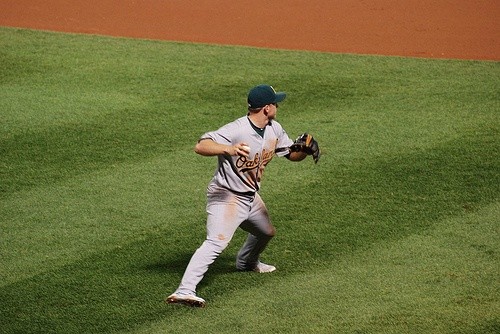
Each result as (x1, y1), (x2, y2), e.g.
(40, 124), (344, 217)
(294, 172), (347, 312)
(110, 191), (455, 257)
(243, 145), (250, 151)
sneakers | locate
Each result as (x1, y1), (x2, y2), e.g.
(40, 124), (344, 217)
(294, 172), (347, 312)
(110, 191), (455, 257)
(165, 290), (206, 308)
(241, 259), (277, 274)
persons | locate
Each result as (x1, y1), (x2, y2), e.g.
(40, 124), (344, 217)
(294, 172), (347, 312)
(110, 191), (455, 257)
(166, 84), (319, 309)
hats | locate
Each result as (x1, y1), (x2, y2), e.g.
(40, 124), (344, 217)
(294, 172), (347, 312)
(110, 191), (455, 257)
(247, 85), (288, 106)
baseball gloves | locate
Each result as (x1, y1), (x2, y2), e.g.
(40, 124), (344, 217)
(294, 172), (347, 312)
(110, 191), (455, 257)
(290, 133), (319, 164)
